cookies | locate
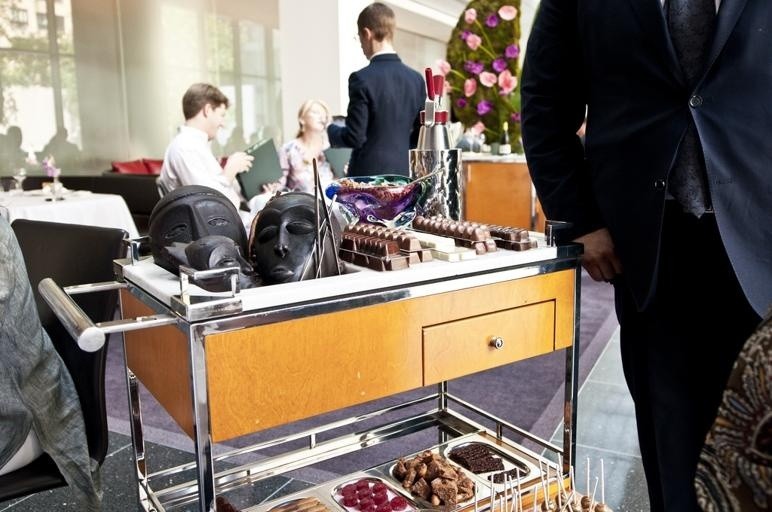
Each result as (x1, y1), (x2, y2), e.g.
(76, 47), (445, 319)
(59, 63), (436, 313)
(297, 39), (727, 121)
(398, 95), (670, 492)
(452, 444), (526, 485)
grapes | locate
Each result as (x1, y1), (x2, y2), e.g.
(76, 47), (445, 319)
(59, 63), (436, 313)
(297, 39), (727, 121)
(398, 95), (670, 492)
(342, 480), (407, 512)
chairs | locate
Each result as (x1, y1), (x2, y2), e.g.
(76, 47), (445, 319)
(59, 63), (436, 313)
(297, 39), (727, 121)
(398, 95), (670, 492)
(0, 216), (128, 512)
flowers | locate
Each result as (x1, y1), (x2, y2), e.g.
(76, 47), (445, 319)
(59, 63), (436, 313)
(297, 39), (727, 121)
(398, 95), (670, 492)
(434, 3), (523, 136)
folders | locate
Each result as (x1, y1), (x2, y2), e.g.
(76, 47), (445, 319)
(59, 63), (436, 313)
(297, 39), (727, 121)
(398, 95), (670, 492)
(324, 147), (353, 179)
(236, 136), (286, 202)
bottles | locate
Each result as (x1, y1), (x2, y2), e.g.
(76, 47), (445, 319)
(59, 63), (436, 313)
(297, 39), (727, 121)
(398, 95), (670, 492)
(497, 119), (513, 155)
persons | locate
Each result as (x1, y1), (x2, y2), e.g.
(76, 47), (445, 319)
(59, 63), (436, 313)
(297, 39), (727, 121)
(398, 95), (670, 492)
(252, 191), (345, 285)
(0, 213), (107, 512)
(277, 97), (338, 207)
(516, 0), (771, 512)
(319, 0), (428, 182)
(147, 184), (250, 275)
(155, 81), (277, 219)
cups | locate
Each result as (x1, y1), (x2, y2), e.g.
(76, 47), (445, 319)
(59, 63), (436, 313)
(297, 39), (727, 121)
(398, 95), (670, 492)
(8, 180), (22, 197)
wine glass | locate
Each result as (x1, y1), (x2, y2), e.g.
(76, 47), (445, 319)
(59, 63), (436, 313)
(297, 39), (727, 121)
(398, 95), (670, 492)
(475, 133), (487, 155)
(12, 166), (28, 195)
(465, 127), (477, 154)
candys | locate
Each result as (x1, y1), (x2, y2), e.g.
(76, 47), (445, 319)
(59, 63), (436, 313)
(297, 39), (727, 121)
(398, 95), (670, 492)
(475, 451), (609, 512)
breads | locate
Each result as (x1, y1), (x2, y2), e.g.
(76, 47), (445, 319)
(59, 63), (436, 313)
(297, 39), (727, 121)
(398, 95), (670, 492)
(392, 450), (473, 510)
(270, 497), (333, 512)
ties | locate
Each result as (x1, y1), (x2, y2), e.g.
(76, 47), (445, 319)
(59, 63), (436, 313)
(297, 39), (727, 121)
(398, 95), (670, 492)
(664, 0), (718, 219)
